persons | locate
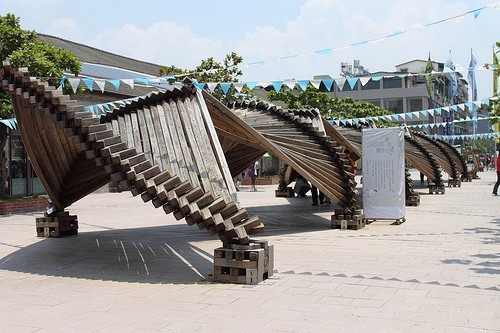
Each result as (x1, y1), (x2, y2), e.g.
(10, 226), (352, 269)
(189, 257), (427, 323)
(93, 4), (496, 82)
(235, 174), (241, 191)
(420, 173), (424, 182)
(312, 185), (331, 206)
(345, 150), (354, 175)
(486, 152), (500, 195)
(251, 161), (259, 192)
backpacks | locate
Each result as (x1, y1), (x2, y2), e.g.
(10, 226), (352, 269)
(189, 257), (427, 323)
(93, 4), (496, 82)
(248, 162), (255, 178)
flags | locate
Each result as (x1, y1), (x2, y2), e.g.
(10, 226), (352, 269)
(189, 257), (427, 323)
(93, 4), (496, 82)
(467, 55), (477, 101)
(424, 57), (434, 97)
(492, 52), (500, 96)
(444, 57), (458, 106)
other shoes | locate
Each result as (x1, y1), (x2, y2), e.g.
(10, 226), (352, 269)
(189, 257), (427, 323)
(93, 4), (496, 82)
(492, 191), (498, 195)
(250, 190), (254, 192)
(235, 186), (239, 192)
(254, 188), (257, 191)
